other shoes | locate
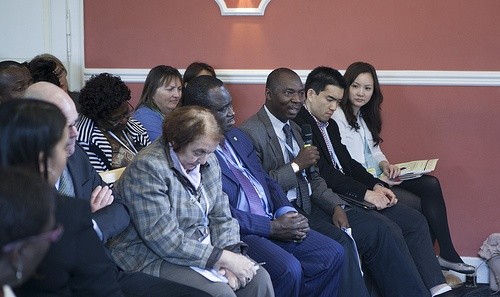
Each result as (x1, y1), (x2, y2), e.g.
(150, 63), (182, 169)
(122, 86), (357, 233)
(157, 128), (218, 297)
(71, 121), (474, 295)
(437, 256), (476, 275)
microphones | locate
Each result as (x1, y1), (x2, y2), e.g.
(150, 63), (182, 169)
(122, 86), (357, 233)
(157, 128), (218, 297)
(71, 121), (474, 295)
(301, 123), (315, 176)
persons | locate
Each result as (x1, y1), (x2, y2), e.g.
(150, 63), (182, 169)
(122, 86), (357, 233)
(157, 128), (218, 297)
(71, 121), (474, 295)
(74, 73), (152, 185)
(129, 65), (182, 143)
(234, 67), (431, 297)
(182, 63), (344, 297)
(106, 105), (277, 297)
(292, 65), (456, 297)
(331, 62), (478, 274)
(0, 52), (213, 297)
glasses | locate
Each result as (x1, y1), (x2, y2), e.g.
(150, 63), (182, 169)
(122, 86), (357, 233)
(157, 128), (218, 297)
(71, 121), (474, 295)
(102, 101), (135, 125)
(3, 223), (63, 253)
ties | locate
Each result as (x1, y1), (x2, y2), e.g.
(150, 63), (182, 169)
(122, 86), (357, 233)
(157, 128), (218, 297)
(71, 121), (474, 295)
(282, 124), (312, 216)
(218, 139), (264, 216)
(57, 166), (76, 199)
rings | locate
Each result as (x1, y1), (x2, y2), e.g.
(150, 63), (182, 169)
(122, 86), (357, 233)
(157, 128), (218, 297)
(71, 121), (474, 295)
(246, 278), (251, 281)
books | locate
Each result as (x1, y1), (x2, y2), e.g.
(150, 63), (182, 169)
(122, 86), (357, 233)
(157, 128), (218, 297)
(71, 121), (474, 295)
(389, 157), (439, 181)
